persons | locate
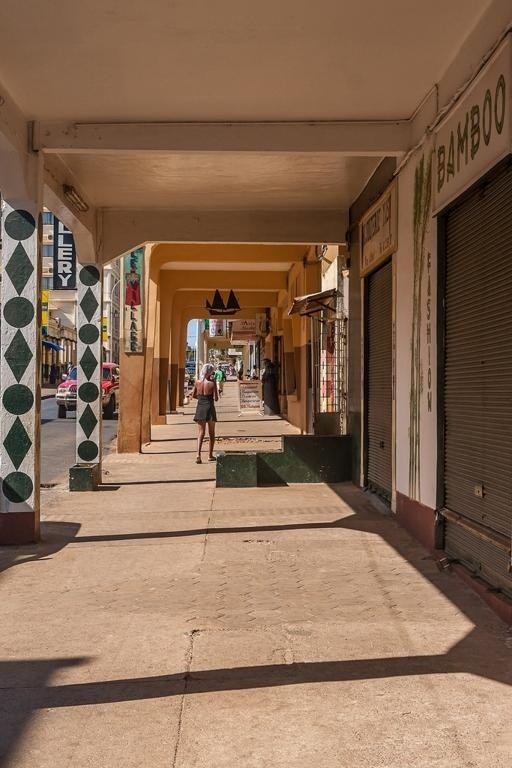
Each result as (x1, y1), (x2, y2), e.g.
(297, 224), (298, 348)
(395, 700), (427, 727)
(192, 362), (280, 463)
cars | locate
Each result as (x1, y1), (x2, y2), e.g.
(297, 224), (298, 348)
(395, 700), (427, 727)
(184, 357), (199, 389)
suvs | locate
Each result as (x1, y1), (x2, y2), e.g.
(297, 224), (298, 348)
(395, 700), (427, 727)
(54, 361), (120, 420)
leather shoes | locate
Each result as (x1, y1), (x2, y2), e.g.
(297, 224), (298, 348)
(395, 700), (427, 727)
(195, 458), (202, 463)
(208, 457), (217, 461)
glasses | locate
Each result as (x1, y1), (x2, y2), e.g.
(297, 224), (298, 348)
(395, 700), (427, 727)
(211, 372), (216, 375)
